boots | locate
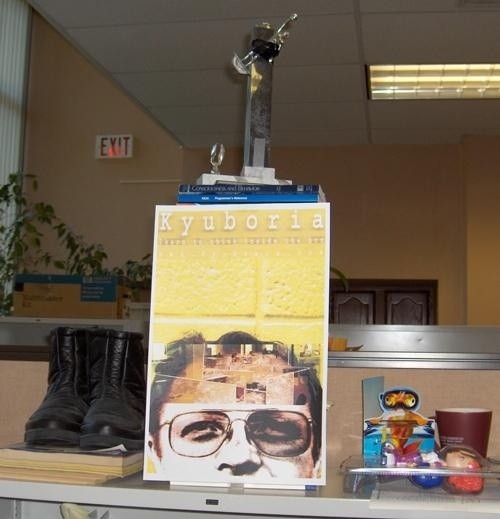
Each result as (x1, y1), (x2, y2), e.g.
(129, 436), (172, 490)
(22, 326), (90, 447)
(79, 326), (145, 451)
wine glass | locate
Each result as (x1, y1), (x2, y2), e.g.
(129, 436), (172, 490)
(210, 143), (225, 175)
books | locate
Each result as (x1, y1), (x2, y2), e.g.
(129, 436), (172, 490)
(1, 458), (143, 478)
(0, 443), (143, 468)
(174, 172), (326, 205)
(0, 467), (116, 486)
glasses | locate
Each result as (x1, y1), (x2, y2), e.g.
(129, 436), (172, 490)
(156, 407), (312, 458)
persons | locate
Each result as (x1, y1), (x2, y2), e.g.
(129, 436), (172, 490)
(146, 331), (321, 480)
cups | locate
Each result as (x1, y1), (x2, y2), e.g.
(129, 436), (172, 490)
(426, 407), (493, 458)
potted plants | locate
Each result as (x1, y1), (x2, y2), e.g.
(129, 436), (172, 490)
(107, 252), (152, 319)
